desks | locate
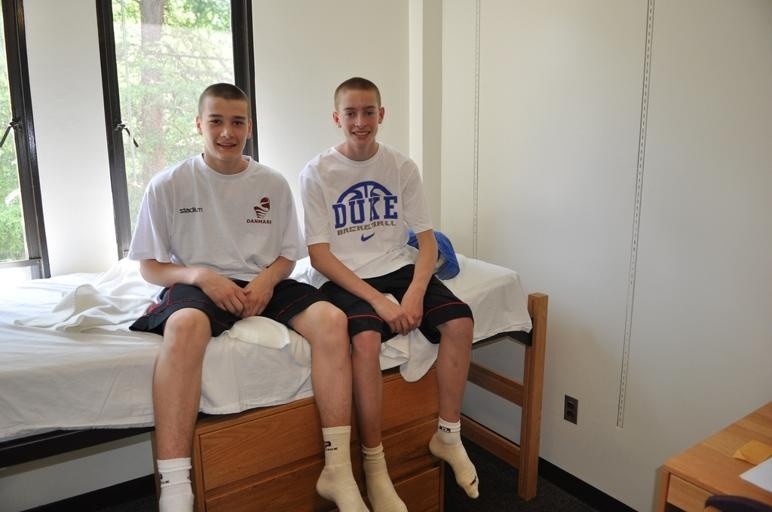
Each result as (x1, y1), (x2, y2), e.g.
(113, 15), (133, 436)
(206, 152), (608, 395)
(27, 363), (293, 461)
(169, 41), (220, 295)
(651, 403), (772, 511)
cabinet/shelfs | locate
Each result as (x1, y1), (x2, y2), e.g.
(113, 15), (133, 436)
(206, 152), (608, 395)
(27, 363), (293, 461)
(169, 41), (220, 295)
(191, 367), (444, 511)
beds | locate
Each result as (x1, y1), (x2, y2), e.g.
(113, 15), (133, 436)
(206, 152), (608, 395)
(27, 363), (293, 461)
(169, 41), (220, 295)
(1, 255), (549, 511)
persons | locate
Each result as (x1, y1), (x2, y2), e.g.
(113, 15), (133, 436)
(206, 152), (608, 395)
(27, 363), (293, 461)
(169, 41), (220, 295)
(128, 83), (368, 512)
(299, 77), (480, 512)
